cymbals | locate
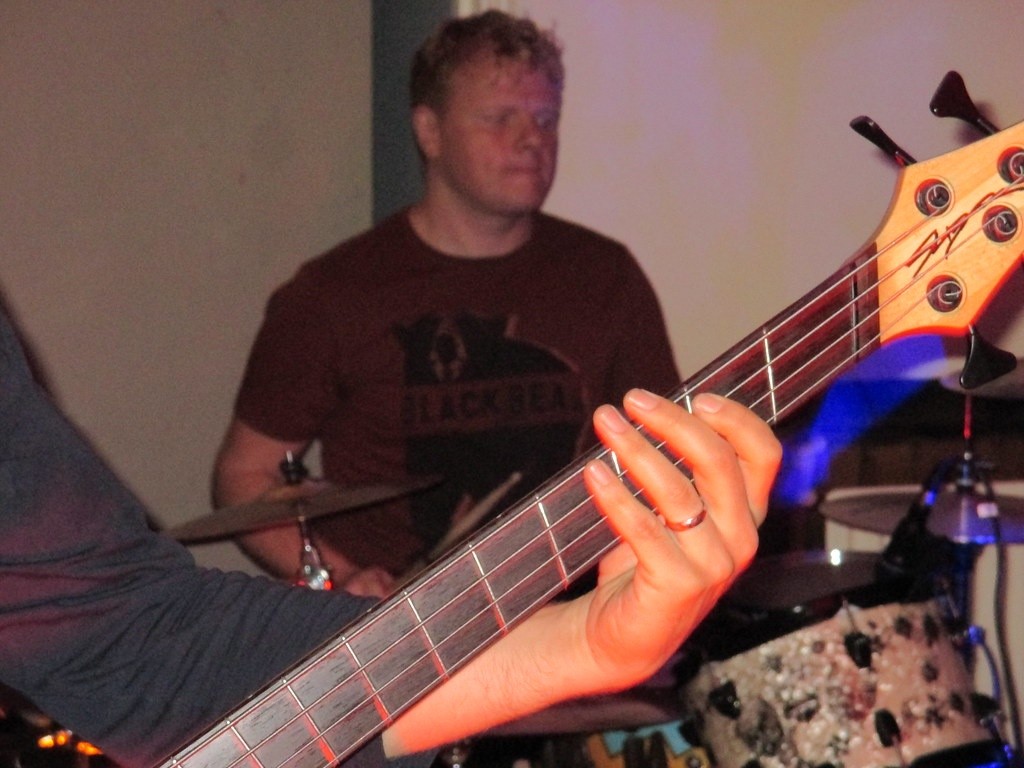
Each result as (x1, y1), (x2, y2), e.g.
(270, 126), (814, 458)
(819, 478), (1023, 546)
(162, 458), (423, 545)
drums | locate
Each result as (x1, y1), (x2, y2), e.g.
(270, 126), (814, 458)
(674, 570), (1009, 768)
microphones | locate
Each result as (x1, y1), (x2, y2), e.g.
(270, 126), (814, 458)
(881, 472), (945, 572)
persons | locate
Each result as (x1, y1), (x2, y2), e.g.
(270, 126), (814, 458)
(0, 286), (786, 768)
(211, 8), (690, 600)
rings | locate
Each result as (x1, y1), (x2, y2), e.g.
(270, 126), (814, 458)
(660, 498), (712, 531)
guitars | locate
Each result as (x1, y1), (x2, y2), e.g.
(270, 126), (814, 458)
(151, 71), (1023, 768)
